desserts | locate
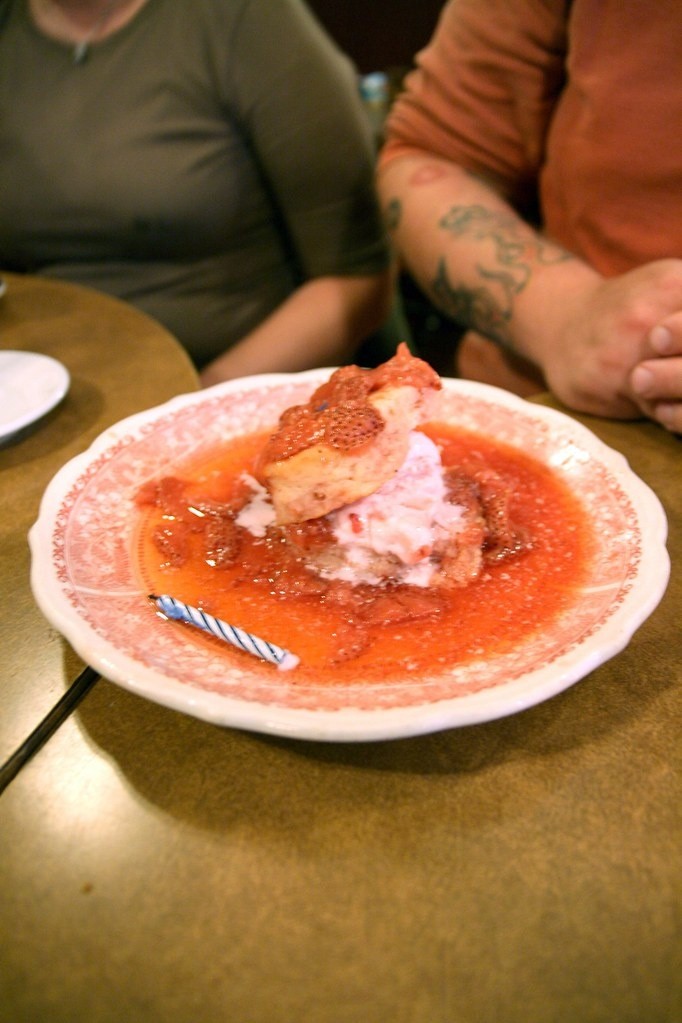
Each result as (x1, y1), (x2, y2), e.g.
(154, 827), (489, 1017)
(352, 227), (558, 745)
(260, 342), (526, 592)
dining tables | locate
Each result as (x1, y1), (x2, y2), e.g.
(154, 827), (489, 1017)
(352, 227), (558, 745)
(0, 273), (682, 1023)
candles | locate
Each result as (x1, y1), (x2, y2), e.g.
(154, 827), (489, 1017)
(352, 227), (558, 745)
(148, 595), (299, 672)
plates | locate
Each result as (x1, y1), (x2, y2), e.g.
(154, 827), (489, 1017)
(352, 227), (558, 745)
(1, 346), (70, 457)
(33, 366), (672, 744)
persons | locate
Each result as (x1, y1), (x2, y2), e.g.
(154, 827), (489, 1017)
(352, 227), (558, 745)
(370, 1), (681, 433)
(0, 0), (392, 390)
